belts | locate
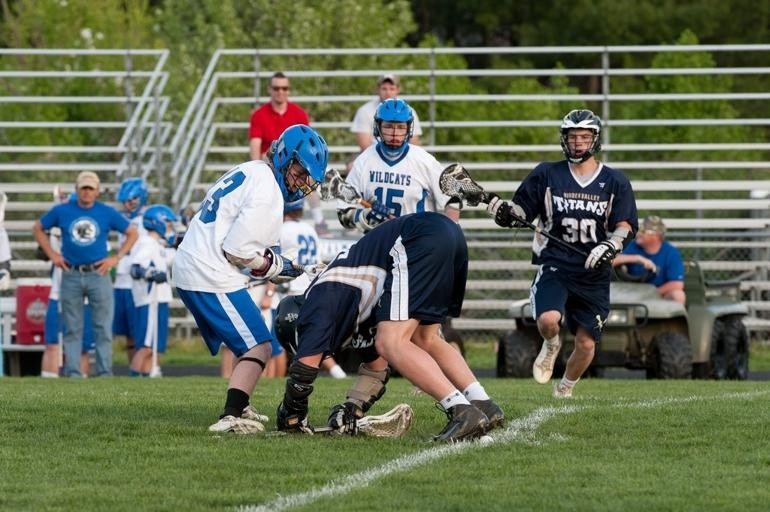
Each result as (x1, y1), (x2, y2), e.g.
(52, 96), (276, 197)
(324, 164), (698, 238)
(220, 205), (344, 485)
(62, 261), (107, 274)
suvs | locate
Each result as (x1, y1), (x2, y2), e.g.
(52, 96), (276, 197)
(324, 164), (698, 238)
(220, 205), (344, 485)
(498, 251), (752, 379)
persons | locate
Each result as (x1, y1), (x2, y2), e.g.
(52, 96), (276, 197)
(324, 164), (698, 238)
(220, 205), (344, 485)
(126, 203), (180, 377)
(350, 73), (423, 152)
(38, 183), (90, 378)
(172, 125), (349, 436)
(112, 175), (150, 376)
(485, 108), (639, 399)
(249, 72), (310, 161)
(336, 97), (461, 222)
(32, 170), (140, 377)
(609, 214), (687, 308)
(274, 211), (505, 442)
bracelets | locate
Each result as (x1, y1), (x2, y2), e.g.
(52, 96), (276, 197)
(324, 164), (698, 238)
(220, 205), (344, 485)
(115, 251), (124, 259)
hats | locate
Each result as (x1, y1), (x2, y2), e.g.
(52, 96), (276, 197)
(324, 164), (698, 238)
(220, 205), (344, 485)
(75, 171), (100, 191)
(378, 73), (400, 86)
(641, 213), (667, 236)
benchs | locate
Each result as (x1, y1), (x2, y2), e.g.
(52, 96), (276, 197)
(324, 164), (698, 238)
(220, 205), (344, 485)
(1, 139), (770, 381)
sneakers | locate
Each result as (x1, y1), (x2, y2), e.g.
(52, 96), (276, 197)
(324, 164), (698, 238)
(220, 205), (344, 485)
(240, 405), (271, 423)
(430, 397), (505, 445)
(531, 338), (561, 386)
(208, 415), (266, 436)
(552, 381), (574, 400)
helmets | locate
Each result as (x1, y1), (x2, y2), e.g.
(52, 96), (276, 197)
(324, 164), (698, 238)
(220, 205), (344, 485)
(117, 177), (149, 219)
(373, 98), (414, 150)
(270, 123), (329, 216)
(558, 109), (606, 165)
(274, 292), (308, 357)
(142, 203), (180, 245)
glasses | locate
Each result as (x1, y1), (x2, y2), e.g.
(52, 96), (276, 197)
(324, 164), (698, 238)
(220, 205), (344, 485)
(271, 85), (288, 91)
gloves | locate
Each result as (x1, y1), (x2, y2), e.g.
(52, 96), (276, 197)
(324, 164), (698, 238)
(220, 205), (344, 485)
(328, 404), (360, 438)
(362, 201), (391, 228)
(274, 402), (313, 437)
(249, 248), (303, 285)
(584, 237), (621, 271)
(485, 192), (528, 231)
